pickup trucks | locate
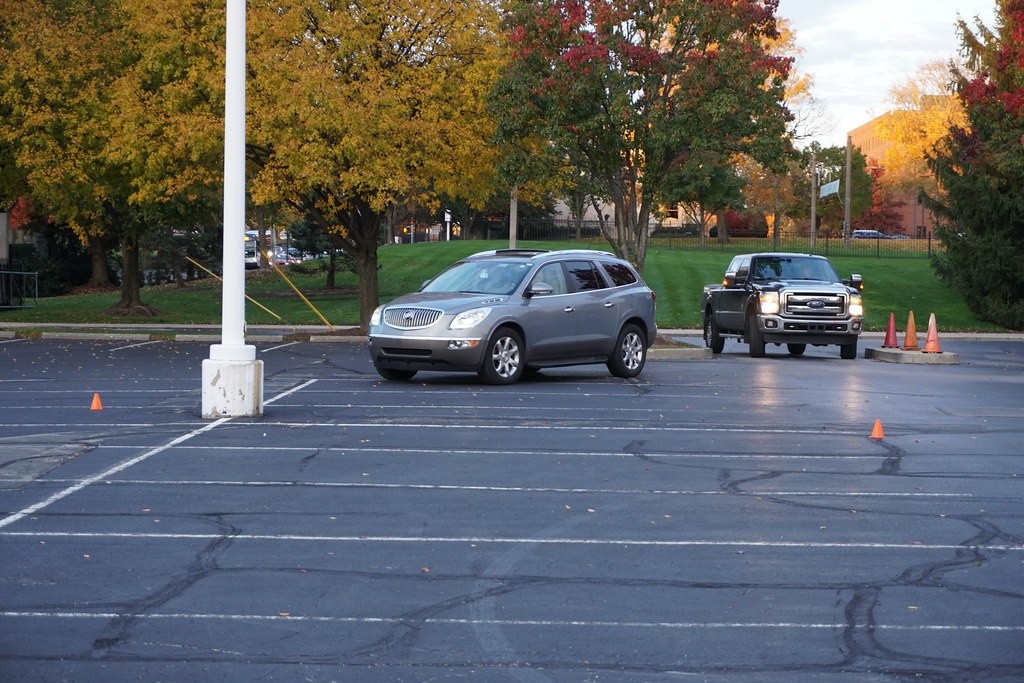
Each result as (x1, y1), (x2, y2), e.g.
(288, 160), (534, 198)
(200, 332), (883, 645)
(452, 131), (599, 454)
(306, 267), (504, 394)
(701, 252), (863, 359)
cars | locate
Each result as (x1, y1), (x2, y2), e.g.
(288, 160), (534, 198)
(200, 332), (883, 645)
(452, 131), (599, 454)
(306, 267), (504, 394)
(853, 229), (885, 239)
(263, 245), (319, 268)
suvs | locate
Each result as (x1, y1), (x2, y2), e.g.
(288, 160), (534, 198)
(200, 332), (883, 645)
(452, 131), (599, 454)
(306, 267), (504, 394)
(367, 247), (658, 385)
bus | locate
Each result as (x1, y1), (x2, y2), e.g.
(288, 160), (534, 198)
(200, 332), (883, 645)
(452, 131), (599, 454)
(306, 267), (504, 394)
(245, 233), (262, 270)
